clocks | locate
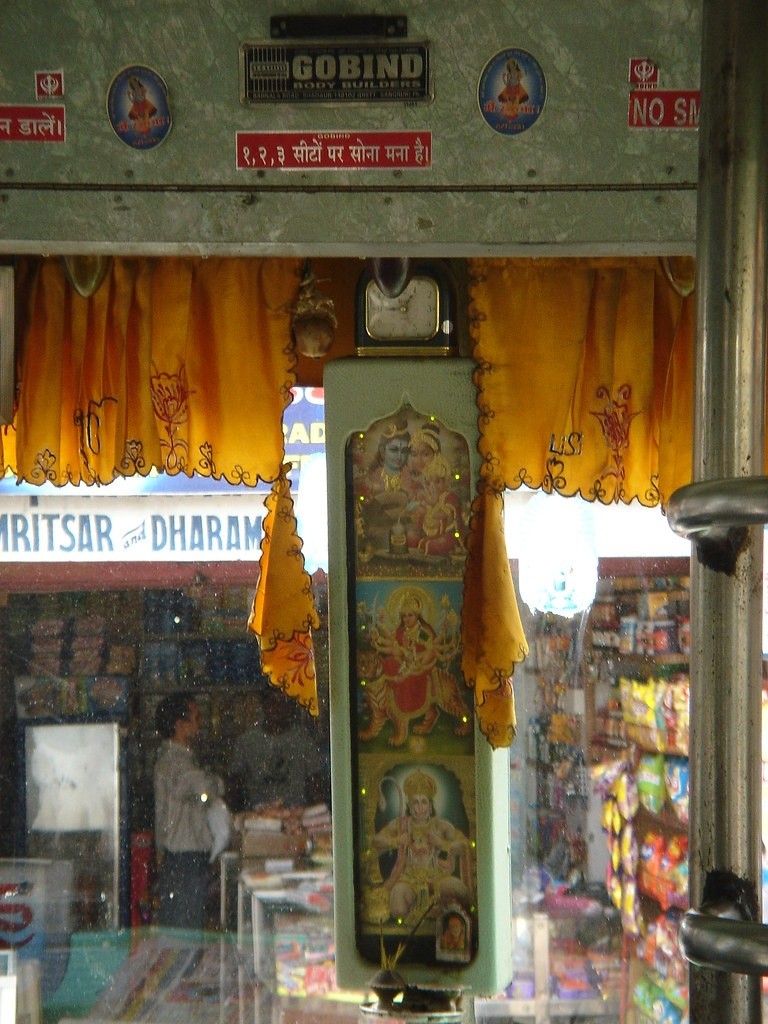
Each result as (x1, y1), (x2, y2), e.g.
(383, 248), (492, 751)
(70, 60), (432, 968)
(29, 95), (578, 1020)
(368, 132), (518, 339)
(354, 267), (457, 357)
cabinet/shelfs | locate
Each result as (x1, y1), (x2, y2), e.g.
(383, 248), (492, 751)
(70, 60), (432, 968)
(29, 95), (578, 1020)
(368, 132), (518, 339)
(0, 570), (692, 1024)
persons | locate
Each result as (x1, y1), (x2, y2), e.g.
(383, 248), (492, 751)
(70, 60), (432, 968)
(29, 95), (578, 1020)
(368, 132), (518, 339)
(227, 685), (327, 810)
(153, 692), (227, 935)
